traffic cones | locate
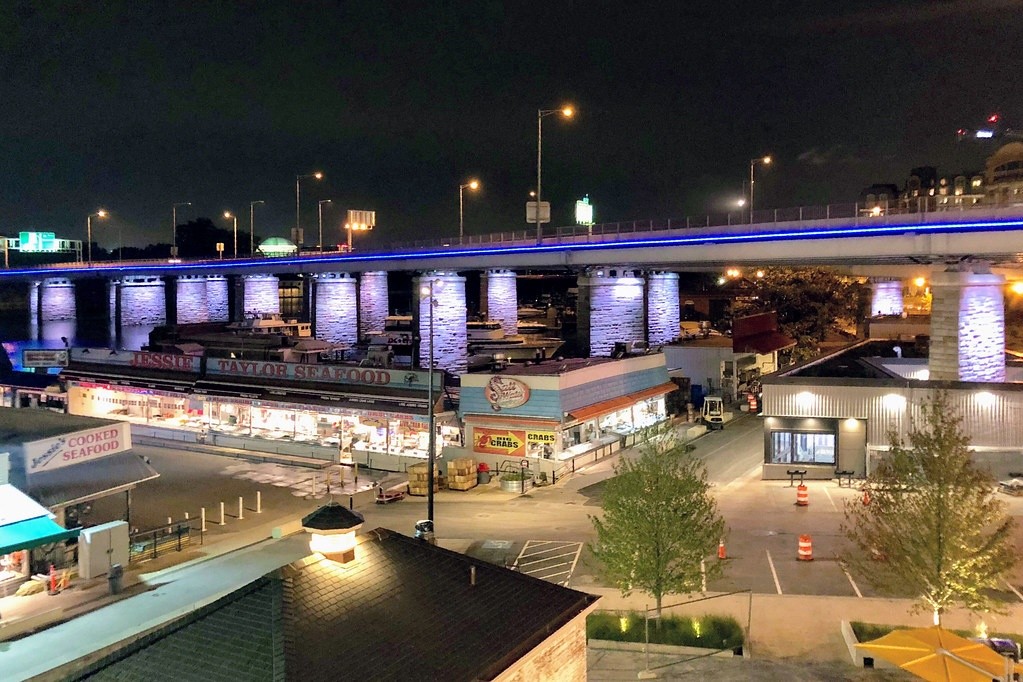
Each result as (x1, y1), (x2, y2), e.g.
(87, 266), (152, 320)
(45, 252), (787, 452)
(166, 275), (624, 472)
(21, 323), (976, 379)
(719, 538), (727, 559)
(47, 564), (60, 597)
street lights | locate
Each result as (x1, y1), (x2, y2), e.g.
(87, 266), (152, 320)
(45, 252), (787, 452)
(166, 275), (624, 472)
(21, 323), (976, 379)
(345, 223), (367, 252)
(225, 213), (237, 259)
(319, 199), (332, 251)
(87, 210), (105, 261)
(460, 182), (478, 244)
(537, 108), (572, 245)
(750, 157), (770, 224)
(251, 201), (264, 257)
(296, 173), (322, 257)
(173, 202), (192, 259)
(738, 200), (745, 224)
(428, 279), (444, 521)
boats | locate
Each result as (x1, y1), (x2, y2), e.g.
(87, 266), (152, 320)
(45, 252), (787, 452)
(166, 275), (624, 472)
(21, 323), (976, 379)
(140, 310), (395, 369)
(365, 307), (567, 367)
